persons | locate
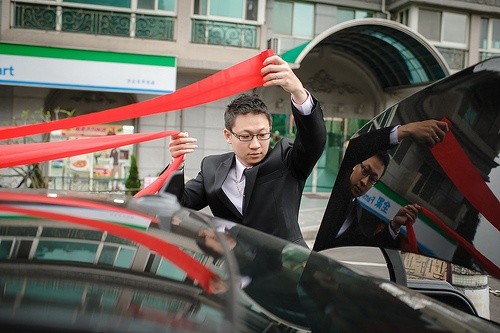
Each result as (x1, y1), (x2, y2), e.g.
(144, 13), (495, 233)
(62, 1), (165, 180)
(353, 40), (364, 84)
(171, 209), (291, 294)
(312, 120), (450, 253)
(158, 55), (327, 250)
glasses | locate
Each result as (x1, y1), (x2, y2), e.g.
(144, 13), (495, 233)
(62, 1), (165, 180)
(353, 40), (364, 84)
(359, 162), (378, 185)
(229, 129), (271, 142)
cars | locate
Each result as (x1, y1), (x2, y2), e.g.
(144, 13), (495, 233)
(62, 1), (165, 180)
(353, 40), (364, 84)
(0, 56), (500, 333)
